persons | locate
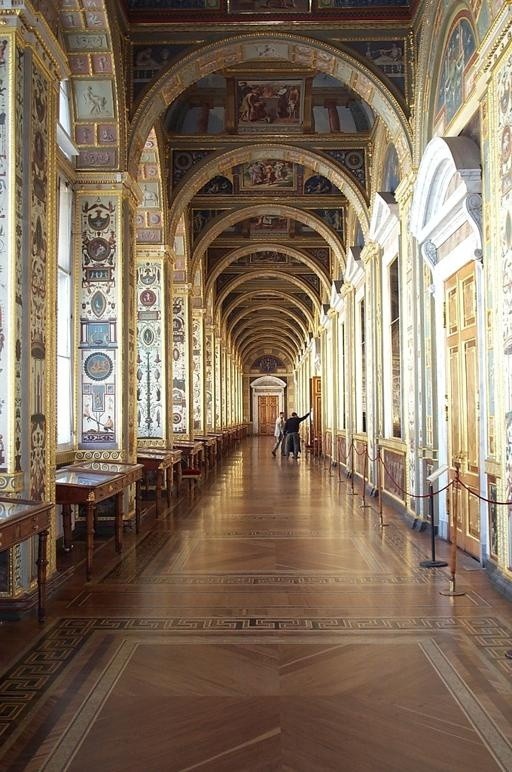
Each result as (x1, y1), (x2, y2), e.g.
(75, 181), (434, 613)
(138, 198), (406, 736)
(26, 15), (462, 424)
(283, 408), (313, 459)
(272, 410), (287, 457)
(235, 80), (299, 263)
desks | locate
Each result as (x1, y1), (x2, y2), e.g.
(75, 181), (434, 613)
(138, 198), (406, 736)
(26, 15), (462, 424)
(0, 496), (56, 618)
(55, 459), (144, 570)
(136, 423), (249, 518)
